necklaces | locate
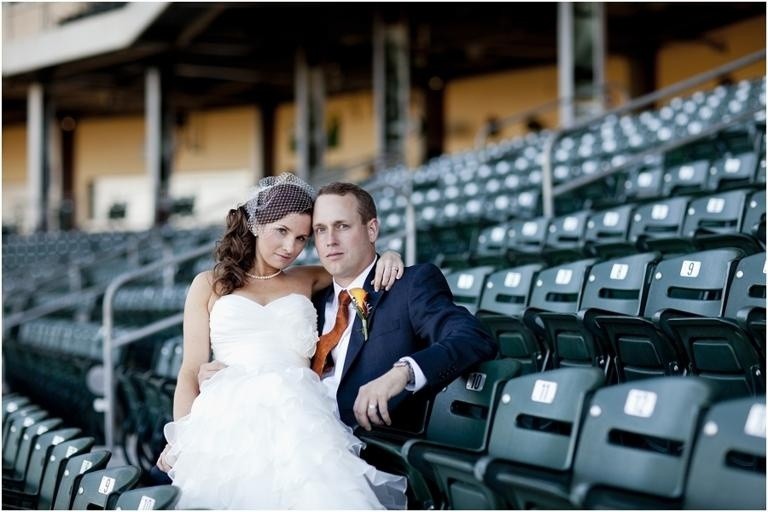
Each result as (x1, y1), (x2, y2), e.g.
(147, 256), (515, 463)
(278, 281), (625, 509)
(244, 270), (282, 280)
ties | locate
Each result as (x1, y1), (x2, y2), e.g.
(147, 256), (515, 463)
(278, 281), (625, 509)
(310, 290), (350, 379)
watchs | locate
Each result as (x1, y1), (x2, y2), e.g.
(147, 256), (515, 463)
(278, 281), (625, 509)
(394, 358), (415, 384)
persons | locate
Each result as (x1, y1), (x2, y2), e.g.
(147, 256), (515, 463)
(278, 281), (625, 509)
(197, 182), (499, 472)
(156, 173), (409, 510)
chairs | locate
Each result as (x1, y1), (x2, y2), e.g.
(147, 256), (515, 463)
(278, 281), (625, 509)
(2, 331), (183, 508)
(351, 148), (768, 509)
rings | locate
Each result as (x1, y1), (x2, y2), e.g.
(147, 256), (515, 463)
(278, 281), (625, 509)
(368, 405), (376, 409)
(391, 266), (399, 271)
